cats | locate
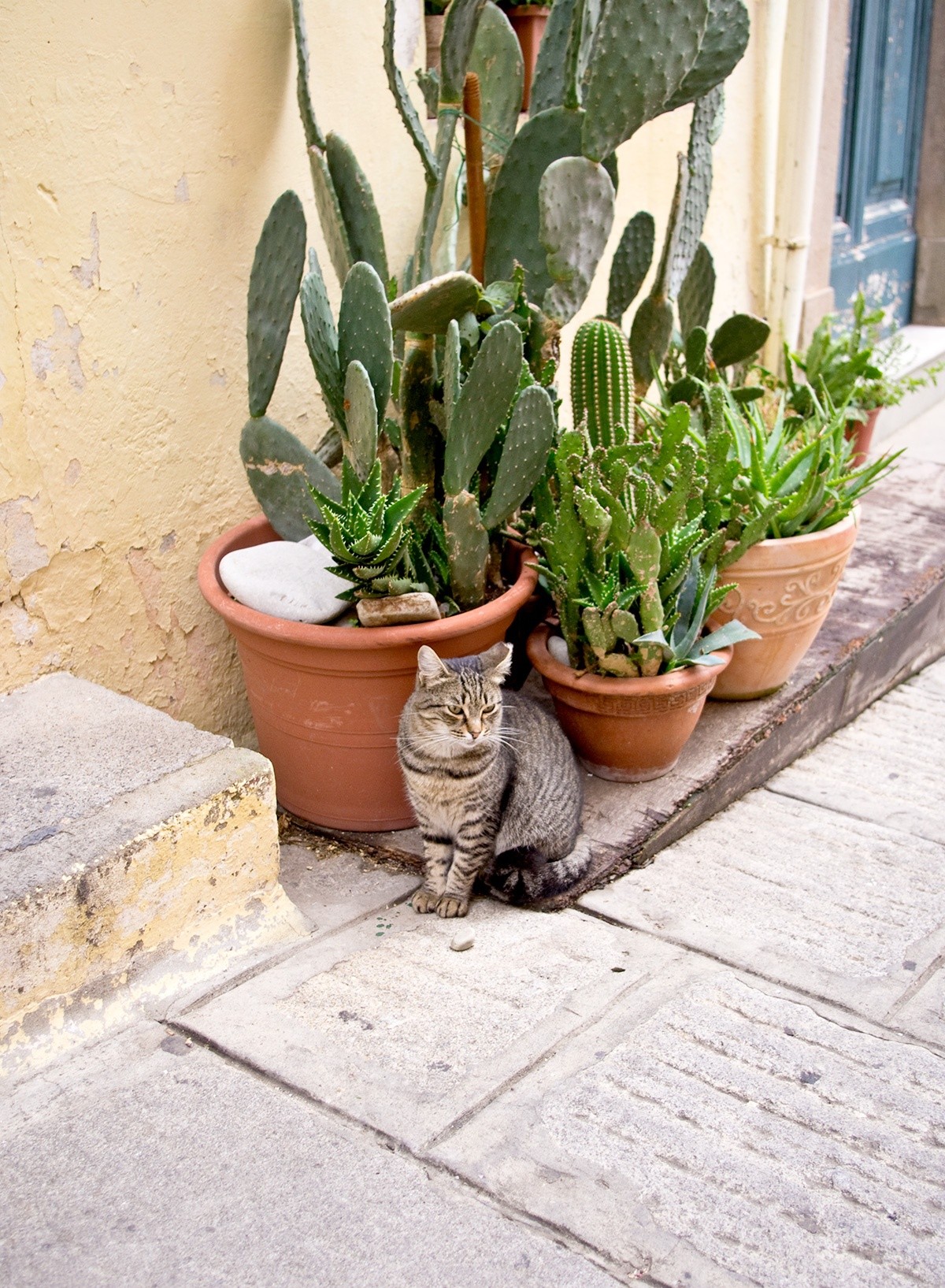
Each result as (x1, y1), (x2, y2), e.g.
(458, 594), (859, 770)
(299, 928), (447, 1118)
(389, 642), (592, 917)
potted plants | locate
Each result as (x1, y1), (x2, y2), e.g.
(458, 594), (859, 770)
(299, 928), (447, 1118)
(193, 0), (945, 837)
